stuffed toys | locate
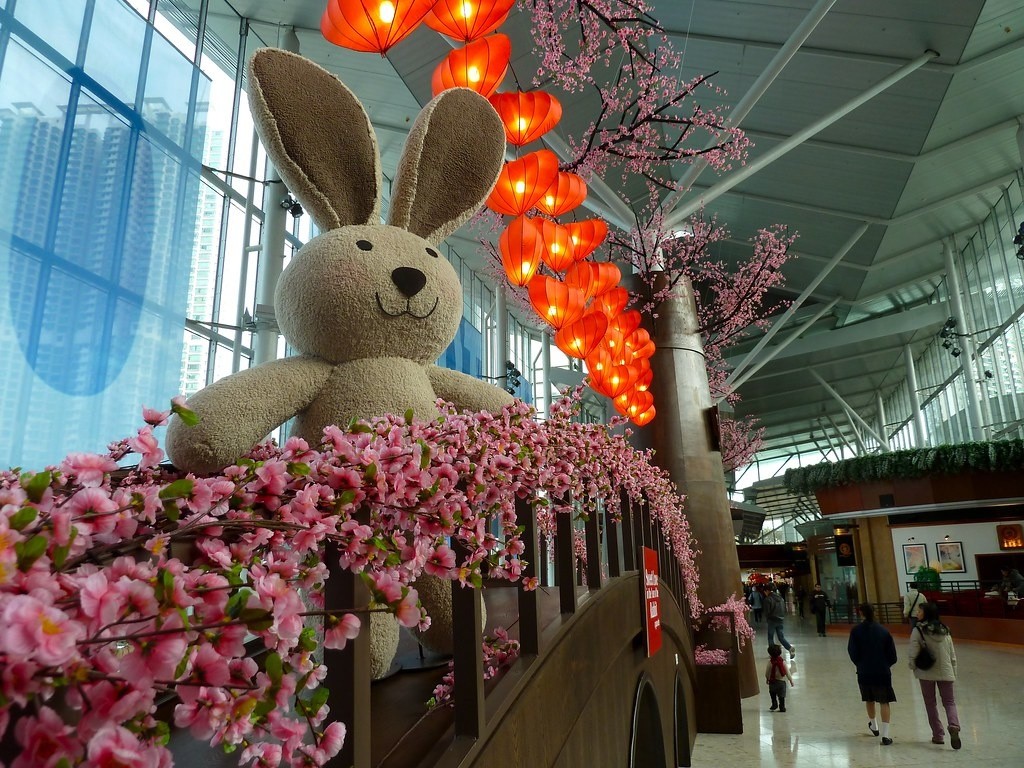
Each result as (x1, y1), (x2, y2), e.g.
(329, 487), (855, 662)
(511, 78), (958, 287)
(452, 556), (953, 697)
(167, 46), (516, 676)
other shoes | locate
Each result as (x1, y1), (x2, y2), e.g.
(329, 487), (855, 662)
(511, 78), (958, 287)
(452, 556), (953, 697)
(931, 737), (944, 744)
(949, 726), (961, 750)
(823, 635), (826, 637)
(781, 708), (786, 712)
(769, 705), (778, 710)
(790, 647), (795, 659)
(819, 634), (821, 636)
(882, 737), (893, 745)
(869, 721), (879, 736)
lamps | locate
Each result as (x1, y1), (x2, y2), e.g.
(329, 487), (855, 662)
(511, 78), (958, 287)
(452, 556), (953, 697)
(290, 199), (304, 218)
(908, 537), (915, 541)
(505, 360), (515, 370)
(940, 317), (1001, 358)
(1012, 221), (1024, 261)
(509, 368), (521, 377)
(984, 370), (993, 379)
(280, 195), (293, 209)
(510, 377), (521, 388)
(945, 535), (949, 540)
(504, 386), (516, 396)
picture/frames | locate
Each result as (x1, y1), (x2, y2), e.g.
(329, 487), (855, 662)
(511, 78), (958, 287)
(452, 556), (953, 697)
(936, 541), (966, 574)
(902, 543), (929, 575)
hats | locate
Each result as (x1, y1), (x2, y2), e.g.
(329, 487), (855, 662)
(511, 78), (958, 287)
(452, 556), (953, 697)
(752, 587), (756, 592)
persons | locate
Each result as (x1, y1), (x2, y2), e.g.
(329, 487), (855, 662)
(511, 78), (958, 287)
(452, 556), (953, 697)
(847, 602), (899, 745)
(794, 585), (808, 618)
(742, 582), (752, 598)
(908, 603), (962, 750)
(809, 584), (832, 637)
(765, 644), (795, 712)
(903, 582), (928, 630)
(1001, 564), (1024, 593)
(762, 585), (795, 660)
(774, 580), (791, 602)
(747, 587), (764, 623)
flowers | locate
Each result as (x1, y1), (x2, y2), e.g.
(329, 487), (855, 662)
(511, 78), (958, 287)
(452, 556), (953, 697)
(1, 0), (800, 768)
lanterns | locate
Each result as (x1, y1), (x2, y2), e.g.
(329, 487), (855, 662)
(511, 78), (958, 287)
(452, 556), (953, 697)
(564, 218), (608, 262)
(499, 213), (543, 288)
(485, 149), (559, 218)
(529, 274), (584, 331)
(321, 0), (439, 58)
(533, 216), (574, 272)
(555, 311), (608, 361)
(534, 171), (587, 218)
(564, 260), (622, 301)
(586, 287), (656, 427)
(423, 0), (516, 44)
(488, 91), (562, 147)
(431, 33), (512, 99)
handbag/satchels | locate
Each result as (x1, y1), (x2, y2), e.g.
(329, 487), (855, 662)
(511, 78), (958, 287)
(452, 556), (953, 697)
(904, 615), (910, 624)
(914, 626), (936, 671)
